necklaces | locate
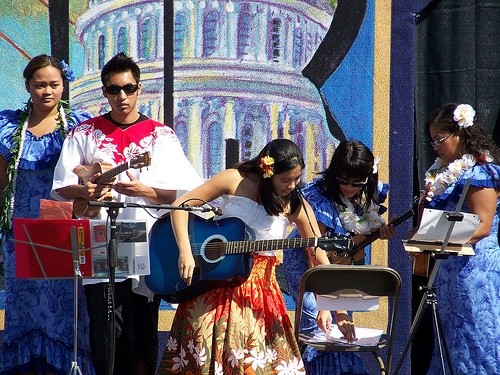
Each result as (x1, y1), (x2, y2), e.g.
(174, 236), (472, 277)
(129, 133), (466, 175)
(424, 151), (494, 201)
(334, 190), (383, 235)
(0, 96), (75, 236)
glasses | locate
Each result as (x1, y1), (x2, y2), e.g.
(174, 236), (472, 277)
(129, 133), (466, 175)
(335, 175), (369, 189)
(430, 132), (453, 148)
(105, 83), (138, 94)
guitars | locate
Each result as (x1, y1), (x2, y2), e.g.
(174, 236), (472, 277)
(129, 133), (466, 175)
(73, 151), (151, 217)
(326, 190), (427, 265)
(144, 211), (355, 304)
(413, 182), (436, 277)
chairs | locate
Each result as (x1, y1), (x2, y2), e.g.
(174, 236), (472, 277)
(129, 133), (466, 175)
(293, 264), (402, 375)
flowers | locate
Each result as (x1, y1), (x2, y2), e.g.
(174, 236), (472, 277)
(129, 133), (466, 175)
(259, 154), (276, 180)
(453, 104), (477, 128)
(371, 155), (380, 176)
(61, 61), (75, 82)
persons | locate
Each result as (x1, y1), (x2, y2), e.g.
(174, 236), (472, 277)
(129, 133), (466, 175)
(50, 50), (204, 375)
(0, 53), (92, 375)
(166, 139), (355, 375)
(287, 138), (396, 375)
(420, 104), (500, 375)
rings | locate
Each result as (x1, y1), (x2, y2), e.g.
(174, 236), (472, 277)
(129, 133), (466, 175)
(384, 234), (388, 237)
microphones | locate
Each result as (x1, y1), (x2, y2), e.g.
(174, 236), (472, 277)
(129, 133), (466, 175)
(212, 207), (223, 216)
